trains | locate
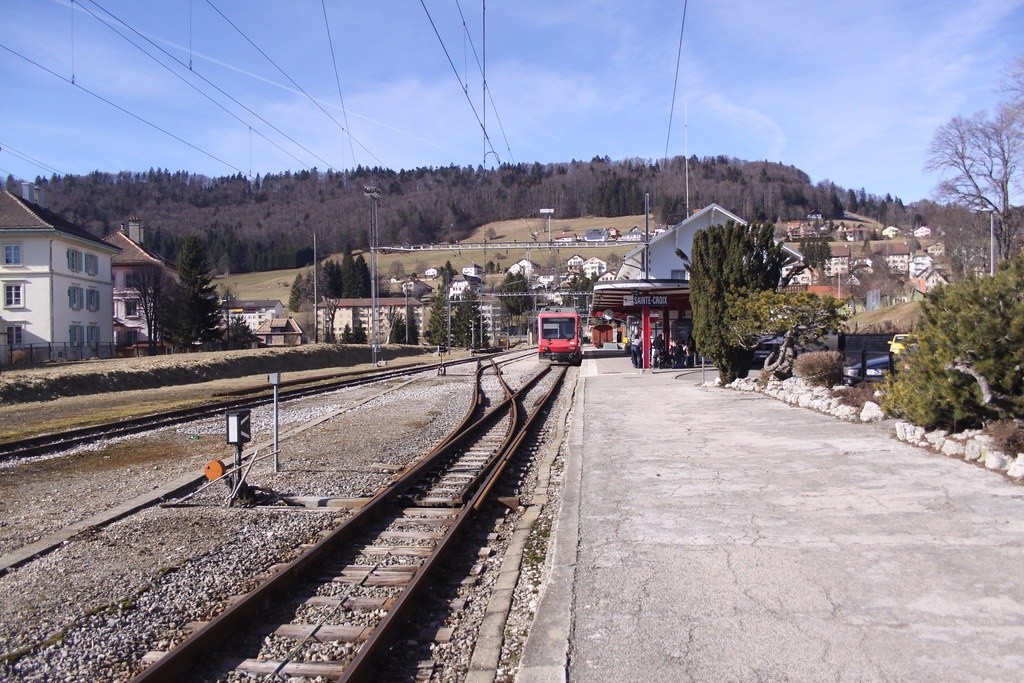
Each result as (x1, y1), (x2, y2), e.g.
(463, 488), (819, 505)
(538, 307), (581, 361)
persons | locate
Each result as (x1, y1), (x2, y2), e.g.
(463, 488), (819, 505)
(630, 326), (696, 370)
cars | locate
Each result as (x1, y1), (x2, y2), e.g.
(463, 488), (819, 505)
(752, 342), (781, 365)
(845, 355), (889, 387)
(888, 333), (917, 354)
